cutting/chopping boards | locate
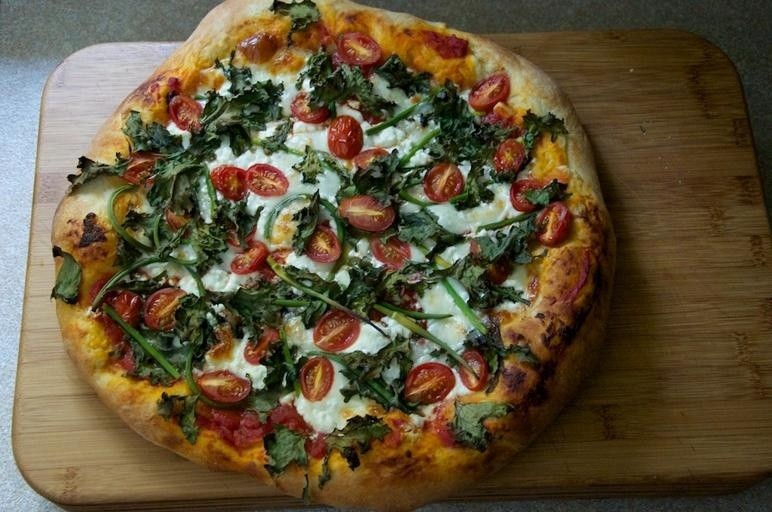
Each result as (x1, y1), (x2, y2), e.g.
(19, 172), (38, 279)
(10, 27), (772, 511)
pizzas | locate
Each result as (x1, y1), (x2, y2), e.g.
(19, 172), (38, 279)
(51, 0), (618, 512)
